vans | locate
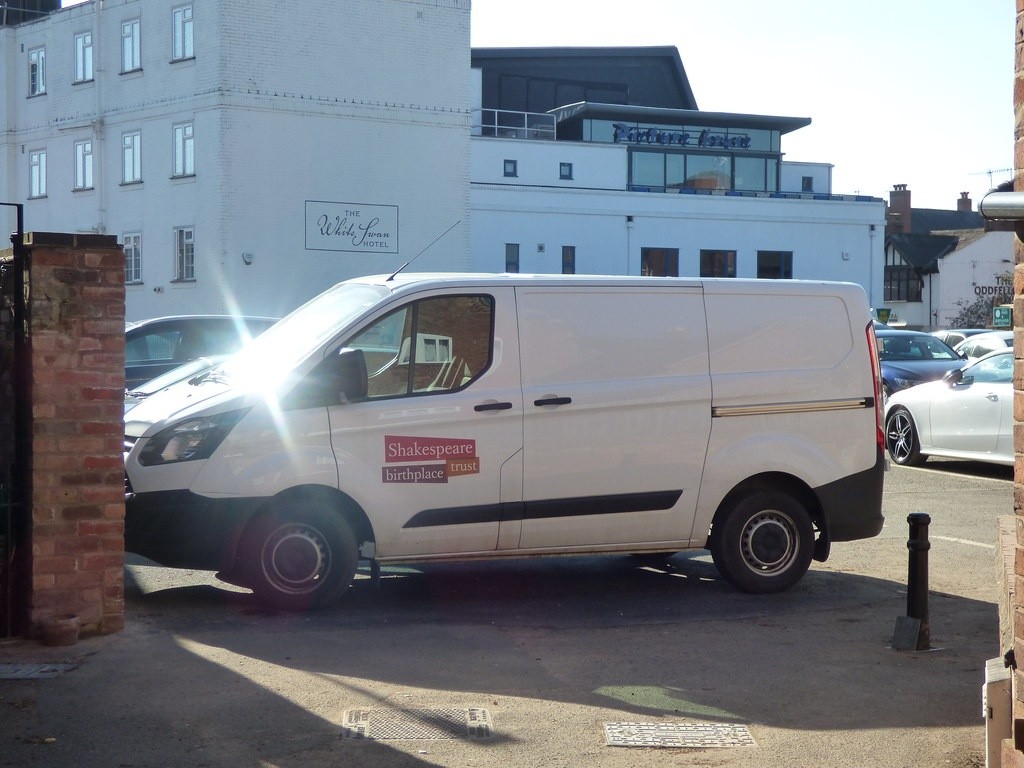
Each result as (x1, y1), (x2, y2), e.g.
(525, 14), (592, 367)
(118, 271), (887, 619)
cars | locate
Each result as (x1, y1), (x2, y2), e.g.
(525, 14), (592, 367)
(871, 318), (1014, 404)
(883, 346), (1015, 470)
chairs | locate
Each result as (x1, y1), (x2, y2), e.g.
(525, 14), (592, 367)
(884, 339), (911, 360)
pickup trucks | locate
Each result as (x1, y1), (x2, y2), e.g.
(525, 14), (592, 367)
(123, 314), (402, 402)
